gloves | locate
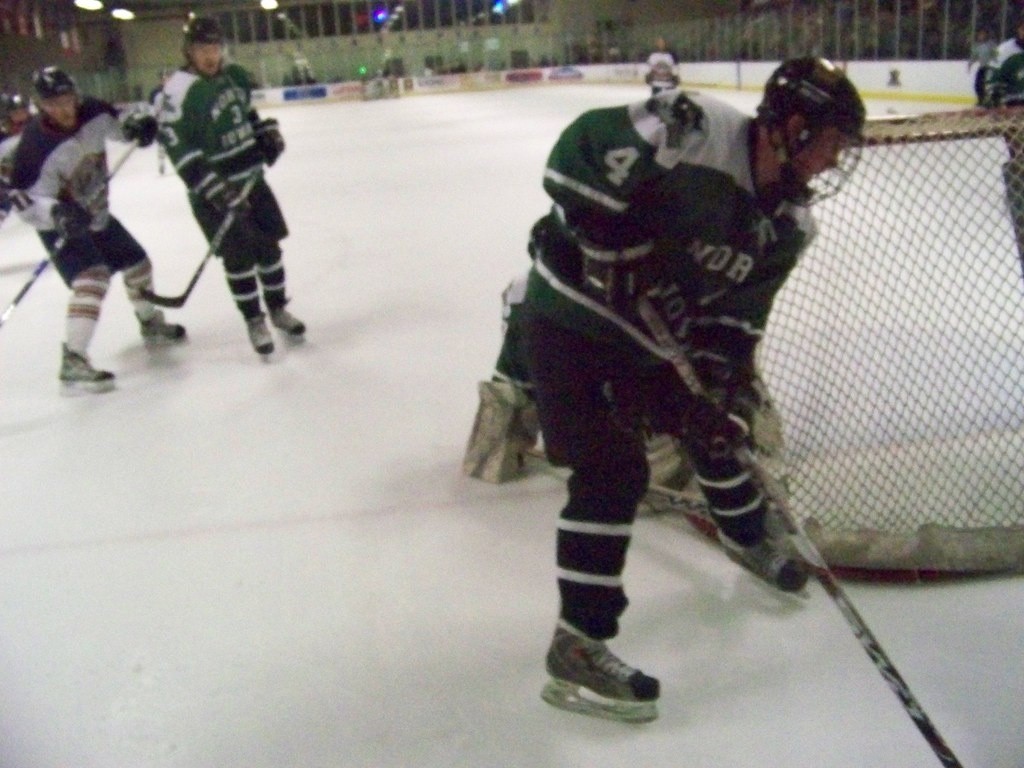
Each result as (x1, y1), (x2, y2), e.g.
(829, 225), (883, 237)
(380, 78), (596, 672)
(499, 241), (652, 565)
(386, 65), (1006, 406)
(51, 202), (92, 242)
(579, 222), (669, 302)
(252, 119), (284, 167)
(123, 116), (158, 147)
(689, 378), (751, 463)
(210, 189), (252, 227)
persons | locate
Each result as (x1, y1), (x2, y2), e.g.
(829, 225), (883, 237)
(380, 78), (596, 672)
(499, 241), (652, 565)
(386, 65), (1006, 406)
(466, 259), (794, 518)
(282, 52), (318, 87)
(154, 14), (307, 357)
(6, 66), (185, 383)
(520, 56), (869, 703)
(149, 69), (177, 176)
(974, 9), (1024, 284)
(1, 92), (40, 222)
(644, 51), (683, 96)
(967, 26), (997, 107)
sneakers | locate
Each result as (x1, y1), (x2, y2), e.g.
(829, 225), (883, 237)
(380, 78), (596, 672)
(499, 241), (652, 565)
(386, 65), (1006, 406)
(717, 529), (812, 601)
(59, 344), (116, 393)
(540, 623), (660, 724)
(137, 310), (186, 343)
(244, 311), (275, 363)
(270, 298), (307, 343)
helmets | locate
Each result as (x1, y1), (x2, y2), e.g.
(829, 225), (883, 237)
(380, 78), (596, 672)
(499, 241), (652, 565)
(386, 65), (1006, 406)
(757, 58), (865, 206)
(5, 92), (28, 111)
(181, 15), (223, 45)
(32, 67), (73, 98)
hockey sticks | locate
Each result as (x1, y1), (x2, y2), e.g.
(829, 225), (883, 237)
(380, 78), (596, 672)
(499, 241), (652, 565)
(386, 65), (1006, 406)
(139, 154), (265, 309)
(2, 136), (140, 326)
(639, 291), (964, 768)
